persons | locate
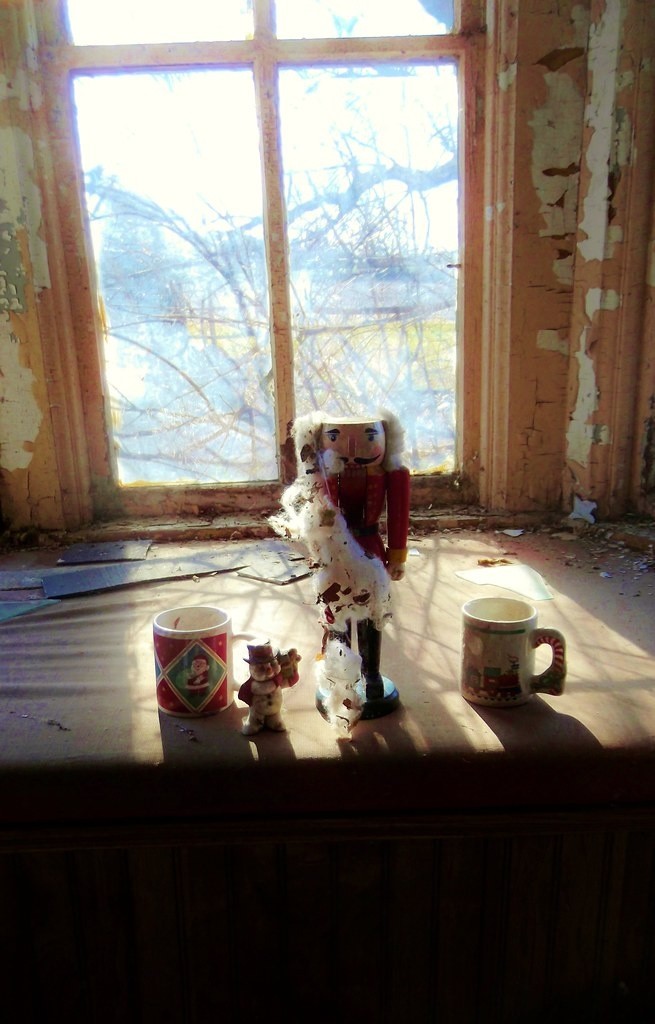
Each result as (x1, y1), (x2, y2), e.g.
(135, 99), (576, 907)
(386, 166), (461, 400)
(318, 410), (412, 723)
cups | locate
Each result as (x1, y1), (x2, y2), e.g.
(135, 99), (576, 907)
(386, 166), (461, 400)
(461, 597), (566, 709)
(153, 606), (259, 717)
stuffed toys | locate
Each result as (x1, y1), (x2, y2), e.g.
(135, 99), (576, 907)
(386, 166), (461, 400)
(238, 637), (301, 736)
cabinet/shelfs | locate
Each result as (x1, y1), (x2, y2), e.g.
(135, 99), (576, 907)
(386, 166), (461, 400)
(0, 797), (648, 1023)
